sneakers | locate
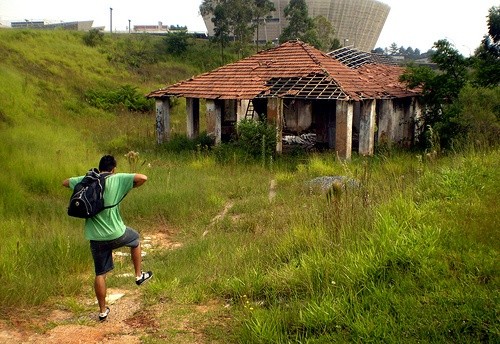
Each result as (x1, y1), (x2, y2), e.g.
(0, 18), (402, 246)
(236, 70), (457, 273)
(99, 306), (110, 321)
(135, 270), (152, 285)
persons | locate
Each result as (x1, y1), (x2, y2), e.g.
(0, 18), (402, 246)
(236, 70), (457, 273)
(63, 154), (152, 320)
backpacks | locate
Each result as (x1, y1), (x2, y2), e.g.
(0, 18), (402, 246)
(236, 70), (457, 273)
(68, 168), (129, 218)
